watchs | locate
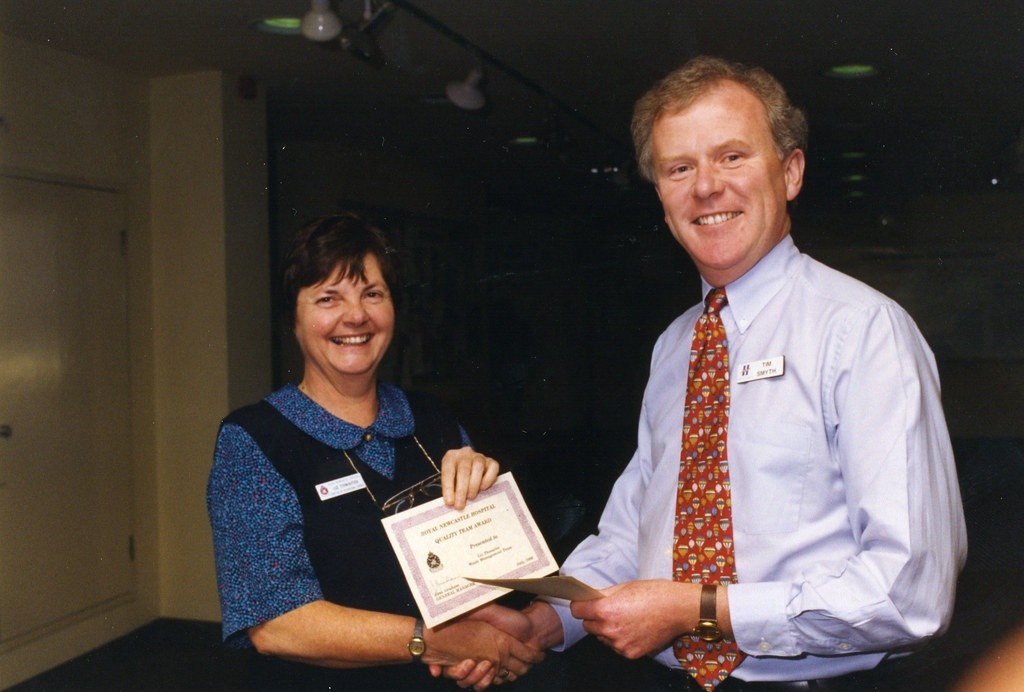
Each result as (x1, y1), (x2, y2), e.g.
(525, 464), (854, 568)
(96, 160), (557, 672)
(407, 619), (425, 662)
(692, 585), (721, 641)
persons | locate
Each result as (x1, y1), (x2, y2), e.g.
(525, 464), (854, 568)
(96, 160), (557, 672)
(207, 207), (546, 692)
(429, 56), (968, 692)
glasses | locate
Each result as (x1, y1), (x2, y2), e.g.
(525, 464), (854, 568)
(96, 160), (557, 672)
(342, 435), (442, 510)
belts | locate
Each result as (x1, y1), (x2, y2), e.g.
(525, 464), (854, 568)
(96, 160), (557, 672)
(747, 674), (848, 692)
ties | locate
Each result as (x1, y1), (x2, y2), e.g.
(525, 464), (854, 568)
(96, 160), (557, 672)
(672, 288), (747, 692)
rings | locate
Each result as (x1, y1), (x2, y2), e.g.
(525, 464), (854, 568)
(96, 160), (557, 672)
(500, 669), (509, 678)
